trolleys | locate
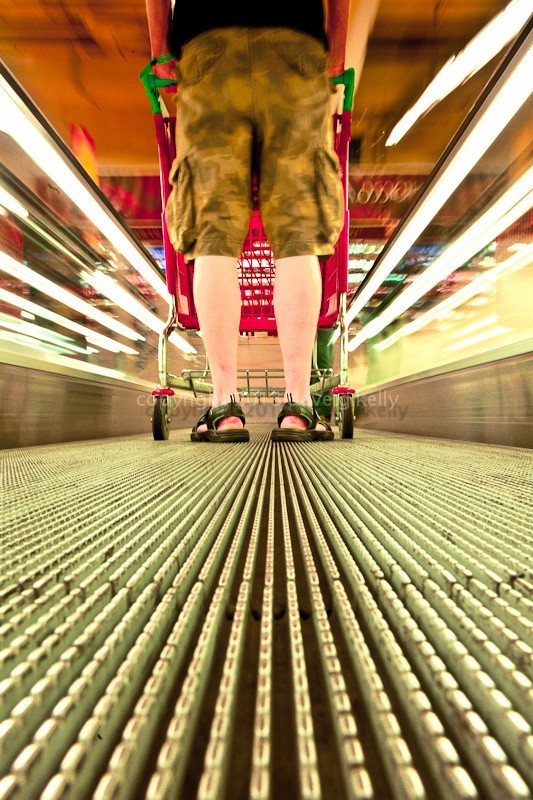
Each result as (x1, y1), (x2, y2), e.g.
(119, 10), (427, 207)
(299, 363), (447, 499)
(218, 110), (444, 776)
(134, 55), (356, 444)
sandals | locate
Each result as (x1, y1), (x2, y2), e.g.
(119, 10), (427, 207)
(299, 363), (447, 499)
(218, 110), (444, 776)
(189, 394), (250, 441)
(269, 393), (333, 441)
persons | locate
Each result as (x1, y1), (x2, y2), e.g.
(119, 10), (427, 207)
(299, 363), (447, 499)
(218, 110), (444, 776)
(144, 0), (349, 442)
(312, 326), (334, 426)
